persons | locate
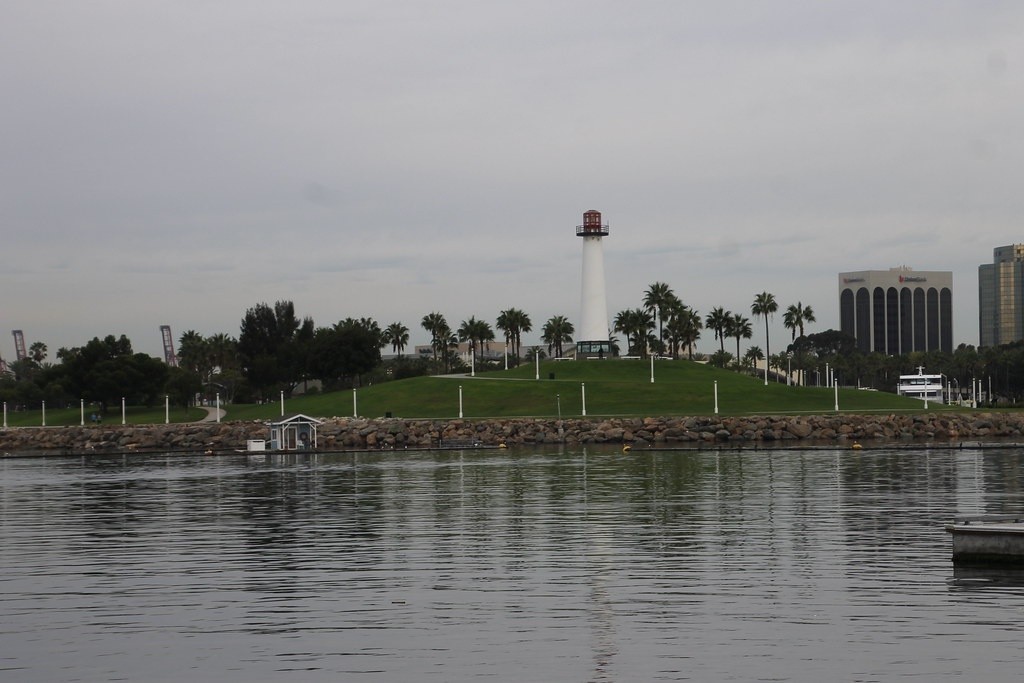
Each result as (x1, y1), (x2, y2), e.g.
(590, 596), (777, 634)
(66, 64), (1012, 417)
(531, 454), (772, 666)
(597, 348), (603, 358)
(91, 413), (102, 425)
